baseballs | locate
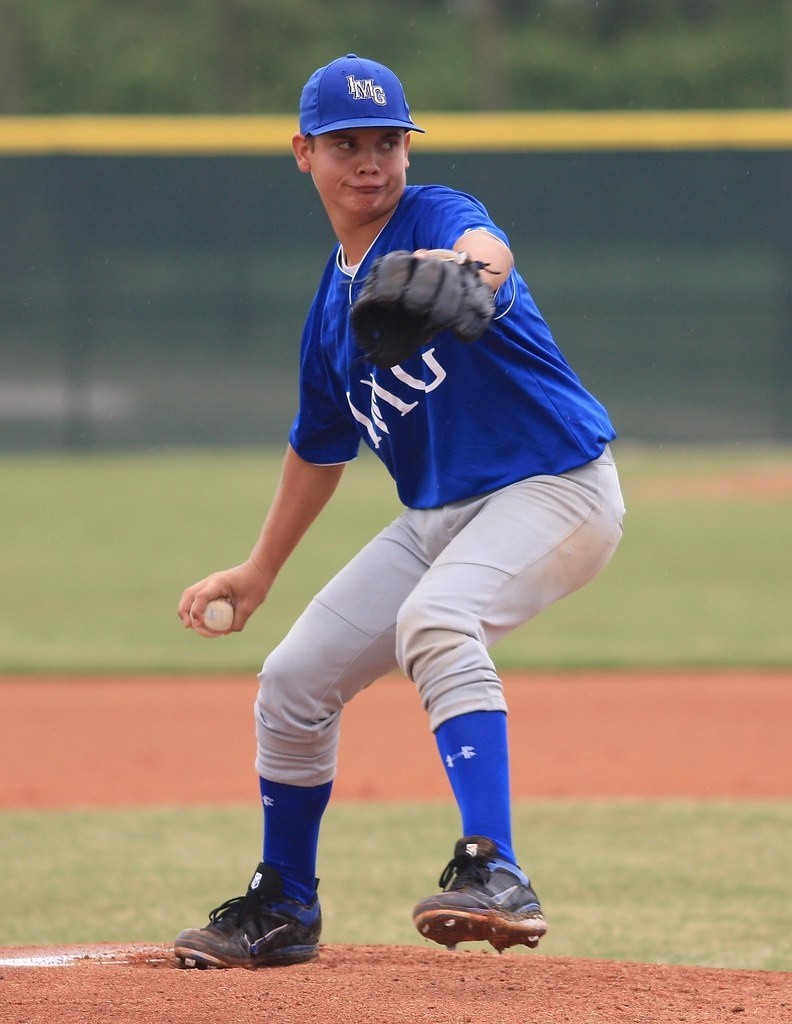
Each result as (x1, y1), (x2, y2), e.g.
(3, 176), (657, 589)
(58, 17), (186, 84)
(202, 599), (234, 632)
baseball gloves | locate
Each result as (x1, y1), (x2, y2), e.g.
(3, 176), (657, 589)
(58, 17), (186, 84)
(354, 249), (492, 367)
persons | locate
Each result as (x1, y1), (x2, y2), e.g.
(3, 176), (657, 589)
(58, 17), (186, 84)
(170, 54), (627, 971)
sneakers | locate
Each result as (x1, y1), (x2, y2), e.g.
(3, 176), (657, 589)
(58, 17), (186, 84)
(175, 862), (322, 969)
(412, 836), (547, 949)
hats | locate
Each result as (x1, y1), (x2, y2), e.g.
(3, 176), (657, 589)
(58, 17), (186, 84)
(297, 54), (426, 136)
